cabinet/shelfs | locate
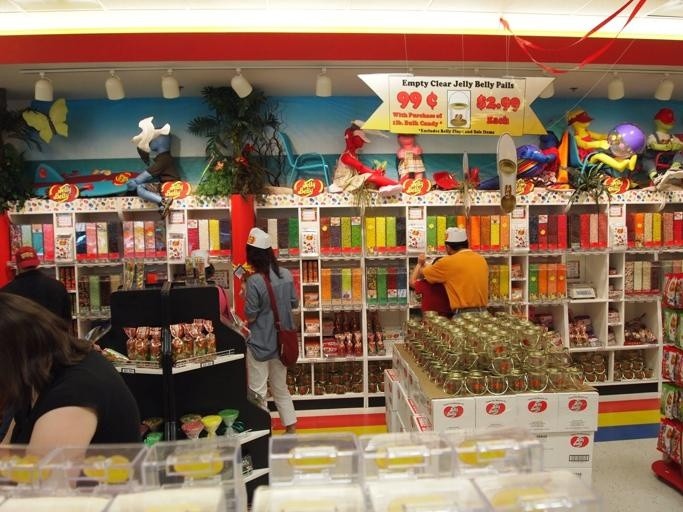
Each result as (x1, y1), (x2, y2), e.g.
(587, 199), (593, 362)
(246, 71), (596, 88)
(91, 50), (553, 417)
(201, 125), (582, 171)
(9, 203), (681, 407)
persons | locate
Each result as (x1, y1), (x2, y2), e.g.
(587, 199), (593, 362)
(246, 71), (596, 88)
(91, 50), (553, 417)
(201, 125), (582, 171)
(396, 134), (426, 183)
(568, 108), (638, 172)
(128, 134), (181, 219)
(0, 246), (72, 329)
(642, 108), (683, 190)
(410, 254), (451, 320)
(420, 227), (489, 316)
(475, 131), (560, 189)
(332, 123), (402, 196)
(0, 289), (141, 496)
(239, 227), (298, 436)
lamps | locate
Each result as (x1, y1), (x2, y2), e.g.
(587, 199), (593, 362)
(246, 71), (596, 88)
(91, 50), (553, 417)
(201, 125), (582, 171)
(105, 70), (125, 100)
(608, 71), (624, 101)
(35, 72), (53, 101)
(655, 73), (674, 100)
(315, 69), (331, 97)
(161, 69), (180, 99)
(230, 69), (252, 98)
(537, 69), (554, 99)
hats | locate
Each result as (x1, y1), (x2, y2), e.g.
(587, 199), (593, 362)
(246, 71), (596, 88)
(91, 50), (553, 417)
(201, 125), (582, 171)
(15, 246), (39, 268)
(246, 227), (272, 249)
(443, 227), (469, 243)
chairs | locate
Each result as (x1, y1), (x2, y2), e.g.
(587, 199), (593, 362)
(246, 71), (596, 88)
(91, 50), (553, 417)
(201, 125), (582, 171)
(275, 129), (329, 187)
(649, 132), (683, 187)
(568, 130), (614, 184)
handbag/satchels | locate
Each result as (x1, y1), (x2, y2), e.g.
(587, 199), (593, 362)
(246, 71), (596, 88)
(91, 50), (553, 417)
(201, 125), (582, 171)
(257, 270), (300, 367)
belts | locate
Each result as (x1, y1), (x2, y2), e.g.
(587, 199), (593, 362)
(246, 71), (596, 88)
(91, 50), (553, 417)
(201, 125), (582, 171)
(453, 306), (488, 313)
